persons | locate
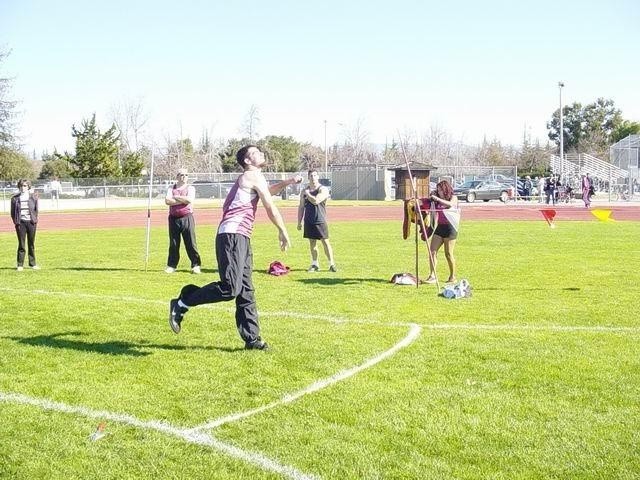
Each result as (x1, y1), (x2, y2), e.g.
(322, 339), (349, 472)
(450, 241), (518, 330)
(165, 169), (201, 274)
(11, 179), (42, 271)
(169, 144), (302, 350)
(587, 173), (594, 201)
(580, 173), (591, 208)
(423, 180), (457, 284)
(50, 178), (62, 201)
(297, 169), (337, 273)
(524, 174), (562, 205)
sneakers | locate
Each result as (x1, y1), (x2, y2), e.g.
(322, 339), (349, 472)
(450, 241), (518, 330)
(424, 276), (436, 283)
(193, 266), (200, 274)
(245, 336), (269, 351)
(169, 299), (188, 333)
(308, 265), (318, 272)
(165, 267), (176, 273)
(330, 265), (336, 272)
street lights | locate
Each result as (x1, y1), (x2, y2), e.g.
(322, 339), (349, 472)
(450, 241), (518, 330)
(557, 79), (565, 177)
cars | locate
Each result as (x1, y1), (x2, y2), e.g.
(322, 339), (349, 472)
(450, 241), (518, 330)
(453, 179), (509, 203)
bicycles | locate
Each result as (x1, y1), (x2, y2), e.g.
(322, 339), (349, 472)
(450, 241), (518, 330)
(608, 184), (636, 205)
